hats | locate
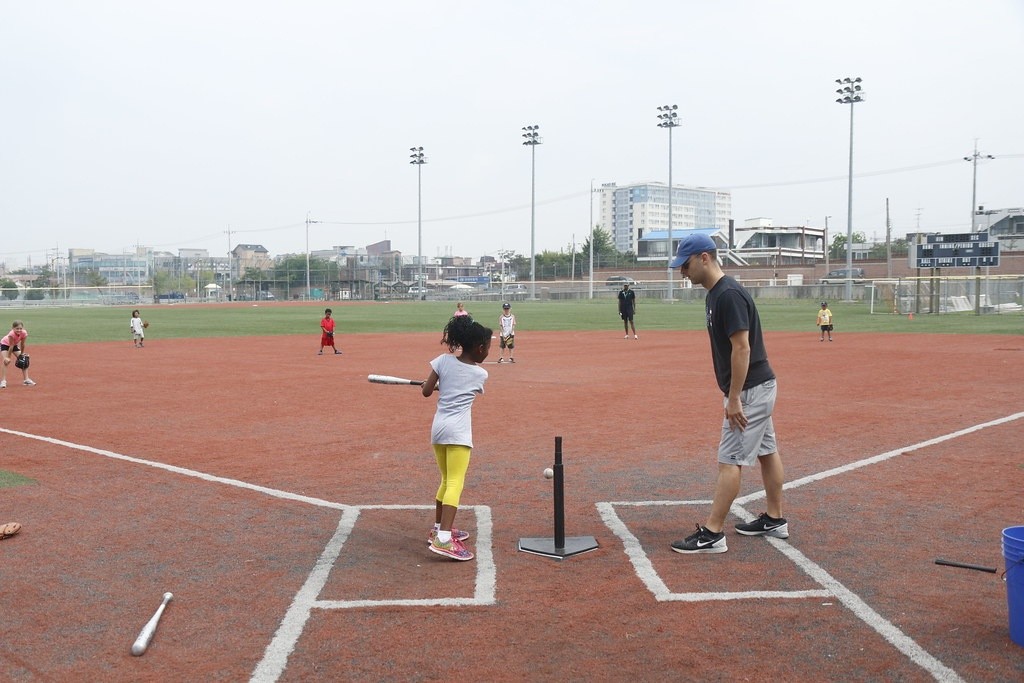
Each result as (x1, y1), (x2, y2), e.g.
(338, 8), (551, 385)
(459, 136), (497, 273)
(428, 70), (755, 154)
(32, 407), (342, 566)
(669, 232), (716, 267)
(502, 303), (510, 308)
(821, 302), (826, 305)
(624, 283), (629, 286)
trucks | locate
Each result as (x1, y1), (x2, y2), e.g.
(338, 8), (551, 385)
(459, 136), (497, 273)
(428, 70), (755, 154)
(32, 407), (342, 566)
(153, 290), (184, 299)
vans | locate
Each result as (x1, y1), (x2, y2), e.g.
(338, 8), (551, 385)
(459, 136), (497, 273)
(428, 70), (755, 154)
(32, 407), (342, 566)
(605, 276), (639, 287)
(257, 291), (274, 300)
(408, 287), (429, 293)
(820, 268), (867, 283)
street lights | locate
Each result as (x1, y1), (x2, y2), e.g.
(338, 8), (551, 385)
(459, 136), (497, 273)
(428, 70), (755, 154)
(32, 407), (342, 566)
(409, 146), (425, 302)
(835, 77), (862, 302)
(825, 214), (832, 274)
(963, 138), (995, 232)
(196, 258), (203, 298)
(522, 125), (540, 302)
(655, 104), (681, 304)
(305, 221), (316, 300)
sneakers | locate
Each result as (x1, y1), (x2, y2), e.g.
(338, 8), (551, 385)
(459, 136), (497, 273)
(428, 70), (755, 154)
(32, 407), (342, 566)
(428, 526), (469, 543)
(429, 537), (474, 561)
(734, 512), (789, 538)
(670, 523), (728, 554)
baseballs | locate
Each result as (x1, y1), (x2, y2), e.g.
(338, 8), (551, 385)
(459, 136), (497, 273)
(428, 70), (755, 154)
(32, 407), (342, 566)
(543, 468), (553, 478)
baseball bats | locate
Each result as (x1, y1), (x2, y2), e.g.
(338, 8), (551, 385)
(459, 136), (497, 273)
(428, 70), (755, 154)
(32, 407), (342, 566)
(367, 373), (437, 387)
(130, 592), (174, 656)
(934, 558), (998, 572)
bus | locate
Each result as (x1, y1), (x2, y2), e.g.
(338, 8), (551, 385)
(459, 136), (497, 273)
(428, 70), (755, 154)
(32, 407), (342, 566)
(445, 276), (493, 288)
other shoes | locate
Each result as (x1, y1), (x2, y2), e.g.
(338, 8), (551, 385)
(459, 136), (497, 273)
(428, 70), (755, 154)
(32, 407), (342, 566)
(498, 358), (504, 363)
(23, 378), (36, 386)
(318, 350), (323, 355)
(509, 358), (513, 362)
(336, 350), (342, 354)
(139, 342), (143, 347)
(634, 335), (638, 339)
(624, 335), (629, 338)
(1, 380), (7, 388)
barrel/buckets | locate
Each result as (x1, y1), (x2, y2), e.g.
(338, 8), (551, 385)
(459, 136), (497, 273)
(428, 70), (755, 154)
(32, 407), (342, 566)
(1001, 526), (1024, 649)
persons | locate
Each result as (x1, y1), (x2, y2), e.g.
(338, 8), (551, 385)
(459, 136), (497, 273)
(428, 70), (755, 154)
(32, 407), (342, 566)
(816, 302), (832, 341)
(417, 312), (493, 561)
(668, 234), (789, 553)
(618, 283), (638, 340)
(454, 302), (467, 316)
(498, 303), (516, 363)
(129, 309), (149, 347)
(0, 320), (36, 388)
(317, 308), (343, 355)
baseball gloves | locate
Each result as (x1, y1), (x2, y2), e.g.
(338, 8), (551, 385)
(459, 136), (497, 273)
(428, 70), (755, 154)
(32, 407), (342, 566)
(327, 331), (335, 337)
(505, 334), (514, 347)
(1, 522), (22, 540)
(827, 324), (833, 331)
(15, 354), (30, 369)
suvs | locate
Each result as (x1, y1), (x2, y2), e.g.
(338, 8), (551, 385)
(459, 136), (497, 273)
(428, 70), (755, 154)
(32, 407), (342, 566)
(500, 284), (526, 292)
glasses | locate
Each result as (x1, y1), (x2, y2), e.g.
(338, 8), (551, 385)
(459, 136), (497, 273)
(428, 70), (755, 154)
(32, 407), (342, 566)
(683, 252), (701, 270)
(504, 308), (509, 309)
